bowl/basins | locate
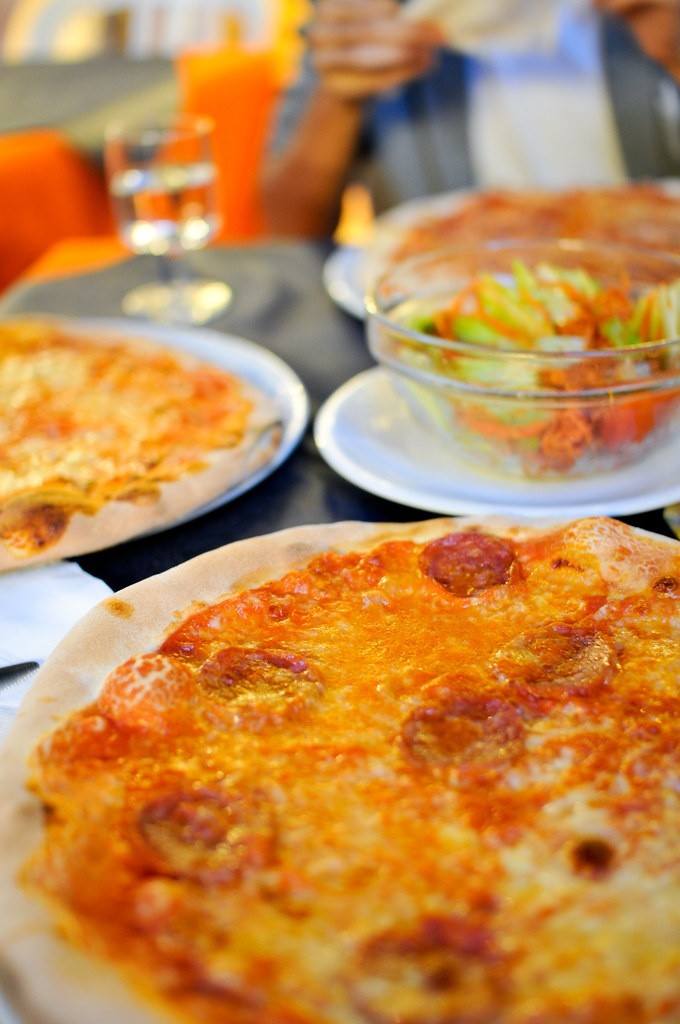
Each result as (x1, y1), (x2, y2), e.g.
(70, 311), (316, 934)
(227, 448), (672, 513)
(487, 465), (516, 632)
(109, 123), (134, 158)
(365, 240), (680, 481)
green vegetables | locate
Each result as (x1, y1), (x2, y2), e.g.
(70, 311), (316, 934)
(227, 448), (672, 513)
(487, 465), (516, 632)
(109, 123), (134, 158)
(395, 258), (679, 428)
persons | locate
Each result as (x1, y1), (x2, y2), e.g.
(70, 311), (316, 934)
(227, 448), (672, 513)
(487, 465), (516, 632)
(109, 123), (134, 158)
(260, 0), (680, 241)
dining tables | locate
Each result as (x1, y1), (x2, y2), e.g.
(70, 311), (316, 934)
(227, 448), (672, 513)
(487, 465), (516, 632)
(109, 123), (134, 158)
(0, 241), (680, 592)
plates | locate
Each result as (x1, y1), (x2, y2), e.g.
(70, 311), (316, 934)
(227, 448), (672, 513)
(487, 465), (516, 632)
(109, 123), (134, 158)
(0, 523), (678, 1024)
(313, 359), (678, 520)
(0, 316), (312, 574)
(321, 231), (417, 317)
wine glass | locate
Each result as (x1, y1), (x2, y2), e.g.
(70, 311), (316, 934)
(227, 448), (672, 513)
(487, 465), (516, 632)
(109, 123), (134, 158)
(100, 112), (235, 329)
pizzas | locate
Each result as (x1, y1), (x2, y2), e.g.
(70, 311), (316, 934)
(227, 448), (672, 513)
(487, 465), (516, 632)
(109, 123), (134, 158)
(0, 513), (680, 1024)
(0, 313), (285, 574)
